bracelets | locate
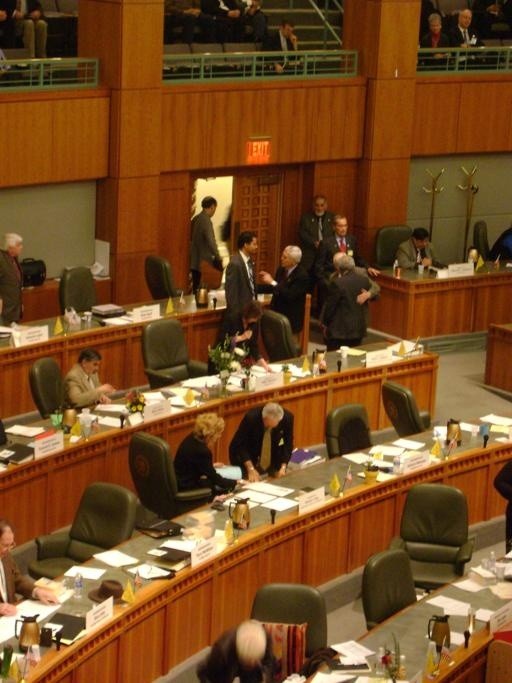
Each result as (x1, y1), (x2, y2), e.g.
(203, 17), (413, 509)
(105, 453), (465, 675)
(247, 467), (254, 470)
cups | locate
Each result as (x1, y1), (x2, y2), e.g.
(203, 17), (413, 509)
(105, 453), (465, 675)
(396, 265), (403, 279)
(38, 626), (53, 646)
(84, 311), (92, 323)
(340, 345), (349, 358)
(479, 420), (490, 437)
(496, 564), (506, 582)
(81, 408), (90, 427)
(471, 426), (479, 437)
(418, 264), (425, 273)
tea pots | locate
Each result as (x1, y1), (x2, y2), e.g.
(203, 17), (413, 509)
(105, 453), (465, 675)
(468, 246), (481, 266)
(426, 612), (452, 650)
(447, 417), (463, 445)
(13, 612), (42, 653)
(56, 401), (80, 433)
(311, 347), (328, 373)
(195, 284), (209, 307)
(228, 496), (251, 530)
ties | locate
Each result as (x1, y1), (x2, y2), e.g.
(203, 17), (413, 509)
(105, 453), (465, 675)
(248, 260), (255, 296)
(260, 430), (272, 471)
(340, 240), (346, 252)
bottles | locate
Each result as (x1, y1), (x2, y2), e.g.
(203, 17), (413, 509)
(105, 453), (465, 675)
(242, 370), (257, 393)
(223, 517), (234, 543)
(375, 635), (409, 680)
(73, 572), (83, 599)
(393, 258), (399, 276)
(430, 432), (441, 457)
(468, 607), (475, 631)
(392, 454), (401, 474)
(488, 551), (497, 572)
(422, 641), (437, 680)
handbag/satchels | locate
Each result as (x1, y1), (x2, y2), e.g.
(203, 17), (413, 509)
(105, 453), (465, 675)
(20, 258), (46, 286)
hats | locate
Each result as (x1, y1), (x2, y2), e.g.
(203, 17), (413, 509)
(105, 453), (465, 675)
(88, 581), (127, 604)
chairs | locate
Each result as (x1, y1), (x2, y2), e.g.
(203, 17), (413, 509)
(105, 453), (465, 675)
(482, 39), (502, 46)
(431, 0), (469, 19)
(502, 39), (512, 47)
(0, 221), (512, 683)
(0, 0), (264, 78)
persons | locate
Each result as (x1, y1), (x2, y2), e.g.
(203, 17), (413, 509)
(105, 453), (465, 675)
(318, 214), (382, 278)
(322, 255), (378, 352)
(0, 232), (24, 324)
(299, 195), (337, 290)
(64, 349), (112, 408)
(0, 518), (61, 617)
(262, 19), (298, 73)
(259, 245), (313, 334)
(448, 9), (485, 64)
(225, 231), (258, 335)
(396, 228), (447, 269)
(172, 413), (245, 505)
(228, 402), (294, 482)
(319, 252), (381, 345)
(199, 0), (241, 18)
(188, 197), (223, 293)
(205, 619), (268, 683)
(5, 0), (48, 58)
(421, 13), (449, 65)
